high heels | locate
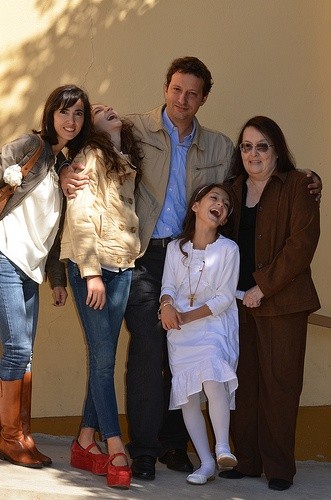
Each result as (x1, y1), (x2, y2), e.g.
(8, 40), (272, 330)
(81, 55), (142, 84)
(108, 453), (131, 485)
(69, 439), (106, 477)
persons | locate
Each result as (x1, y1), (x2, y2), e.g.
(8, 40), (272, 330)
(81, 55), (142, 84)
(0, 85), (92, 468)
(57, 56), (323, 481)
(216, 115), (322, 490)
(157, 182), (241, 485)
(59, 104), (144, 488)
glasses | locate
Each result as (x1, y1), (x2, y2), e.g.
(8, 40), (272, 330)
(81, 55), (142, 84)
(239, 141), (272, 153)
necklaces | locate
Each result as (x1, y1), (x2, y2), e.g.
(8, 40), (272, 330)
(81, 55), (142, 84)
(249, 178), (260, 194)
(187, 261), (206, 307)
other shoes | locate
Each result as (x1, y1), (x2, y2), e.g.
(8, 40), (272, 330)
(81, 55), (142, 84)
(268, 478), (291, 491)
(218, 454), (236, 470)
(219, 465), (263, 479)
(188, 472), (215, 484)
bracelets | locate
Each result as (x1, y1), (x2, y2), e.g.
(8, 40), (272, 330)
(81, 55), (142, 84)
(158, 300), (172, 320)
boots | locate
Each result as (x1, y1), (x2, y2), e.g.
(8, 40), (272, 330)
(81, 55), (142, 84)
(1, 371), (54, 467)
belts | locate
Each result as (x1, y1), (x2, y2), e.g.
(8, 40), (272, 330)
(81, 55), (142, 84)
(150, 238), (171, 247)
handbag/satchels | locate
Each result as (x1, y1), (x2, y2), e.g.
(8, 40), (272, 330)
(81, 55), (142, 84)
(0, 135), (42, 214)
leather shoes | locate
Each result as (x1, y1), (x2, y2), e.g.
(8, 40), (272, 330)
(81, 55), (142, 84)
(162, 454), (193, 473)
(132, 454), (156, 481)
